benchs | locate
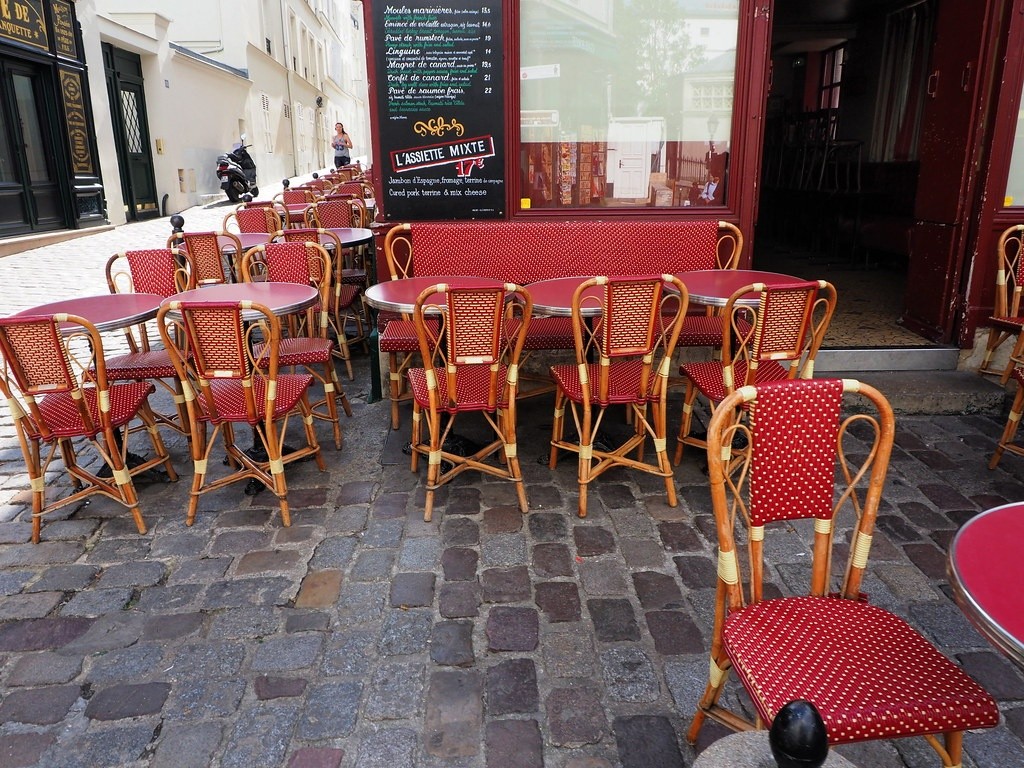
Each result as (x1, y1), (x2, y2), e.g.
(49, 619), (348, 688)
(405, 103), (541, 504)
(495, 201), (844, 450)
(377, 222), (744, 430)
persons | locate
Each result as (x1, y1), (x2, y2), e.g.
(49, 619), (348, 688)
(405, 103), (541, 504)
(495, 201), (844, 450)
(332, 123), (352, 168)
(689, 151), (729, 206)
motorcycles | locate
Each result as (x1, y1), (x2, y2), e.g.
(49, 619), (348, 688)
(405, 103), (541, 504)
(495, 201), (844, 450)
(215, 133), (260, 203)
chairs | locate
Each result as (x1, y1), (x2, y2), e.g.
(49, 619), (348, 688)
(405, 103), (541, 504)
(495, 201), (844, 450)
(686, 379), (1000, 767)
(0, 195), (374, 545)
(763, 107), (884, 269)
(672, 280), (838, 473)
(270, 161), (372, 205)
(549, 277), (690, 518)
(981, 223), (1024, 475)
(409, 283), (532, 521)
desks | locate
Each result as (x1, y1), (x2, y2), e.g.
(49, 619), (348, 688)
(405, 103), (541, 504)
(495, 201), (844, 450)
(176, 233), (279, 283)
(159, 283), (319, 494)
(947, 503), (1024, 672)
(313, 190), (335, 198)
(277, 229), (374, 286)
(6, 293), (181, 520)
(275, 199), (375, 222)
(661, 271), (811, 477)
(516, 275), (660, 470)
(365, 277), (517, 482)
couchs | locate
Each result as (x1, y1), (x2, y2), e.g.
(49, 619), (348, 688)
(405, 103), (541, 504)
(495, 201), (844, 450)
(829, 161), (927, 259)
